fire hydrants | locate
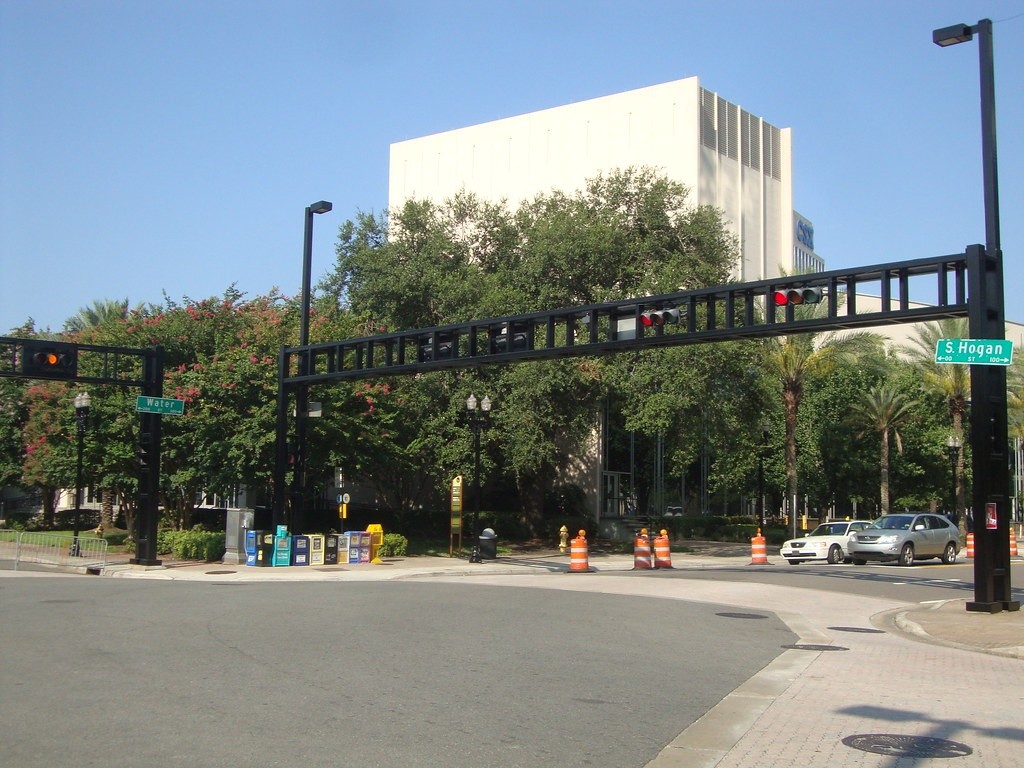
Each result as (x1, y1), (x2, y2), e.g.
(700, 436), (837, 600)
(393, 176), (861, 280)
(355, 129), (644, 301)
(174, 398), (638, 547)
(559, 525), (569, 553)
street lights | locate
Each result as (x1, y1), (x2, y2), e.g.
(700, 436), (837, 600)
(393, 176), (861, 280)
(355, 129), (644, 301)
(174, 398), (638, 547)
(932, 19), (1022, 614)
(68, 392), (91, 556)
(291, 200), (332, 536)
(466, 393), (493, 563)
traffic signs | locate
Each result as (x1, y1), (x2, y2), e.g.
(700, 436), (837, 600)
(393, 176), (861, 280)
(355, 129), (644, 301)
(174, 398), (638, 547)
(935, 339), (1013, 365)
(136, 396), (184, 415)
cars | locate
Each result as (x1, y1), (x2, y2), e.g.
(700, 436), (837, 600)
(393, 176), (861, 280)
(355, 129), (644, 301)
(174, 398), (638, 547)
(780, 519), (883, 565)
(846, 511), (962, 567)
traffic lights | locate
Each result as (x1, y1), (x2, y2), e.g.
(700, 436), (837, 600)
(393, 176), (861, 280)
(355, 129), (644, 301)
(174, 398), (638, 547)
(640, 309), (681, 327)
(772, 286), (821, 306)
(32, 352), (74, 371)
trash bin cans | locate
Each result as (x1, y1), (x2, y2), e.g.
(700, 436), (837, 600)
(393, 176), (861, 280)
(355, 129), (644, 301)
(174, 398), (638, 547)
(479, 528), (497, 559)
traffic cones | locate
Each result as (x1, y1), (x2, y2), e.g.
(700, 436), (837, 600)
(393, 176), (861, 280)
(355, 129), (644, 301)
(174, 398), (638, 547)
(749, 528), (775, 565)
(652, 529), (677, 570)
(628, 528), (654, 571)
(964, 533), (974, 558)
(564, 530), (599, 573)
(1010, 528), (1023, 557)
